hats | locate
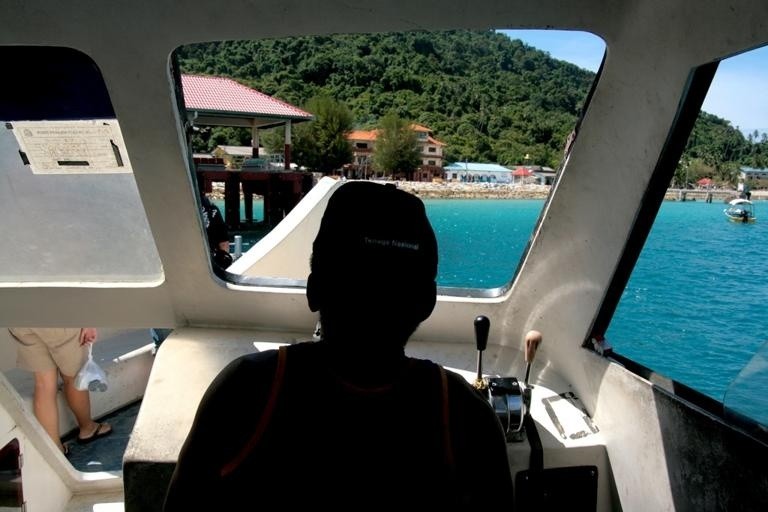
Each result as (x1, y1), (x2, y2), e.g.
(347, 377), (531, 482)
(311, 180), (439, 272)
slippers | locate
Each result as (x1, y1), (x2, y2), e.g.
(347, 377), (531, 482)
(76, 422), (113, 446)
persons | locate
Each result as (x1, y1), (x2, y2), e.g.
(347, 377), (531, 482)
(160, 181), (515, 512)
(7, 327), (113, 459)
(742, 184), (751, 200)
(149, 170), (233, 353)
(740, 210), (749, 223)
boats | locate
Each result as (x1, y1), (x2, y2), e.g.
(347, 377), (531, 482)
(722, 197), (758, 225)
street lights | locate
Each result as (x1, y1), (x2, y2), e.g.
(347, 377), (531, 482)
(524, 152), (532, 166)
